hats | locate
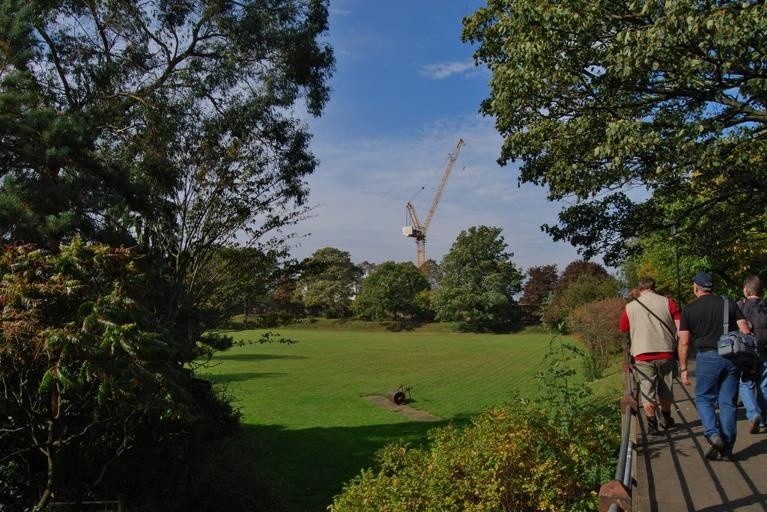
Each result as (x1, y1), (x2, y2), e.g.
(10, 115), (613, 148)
(692, 272), (715, 288)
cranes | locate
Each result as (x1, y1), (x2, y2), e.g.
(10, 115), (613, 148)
(403, 137), (464, 268)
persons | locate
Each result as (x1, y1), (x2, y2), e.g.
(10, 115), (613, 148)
(619, 276), (682, 435)
(736, 276), (767, 434)
(678, 272), (752, 461)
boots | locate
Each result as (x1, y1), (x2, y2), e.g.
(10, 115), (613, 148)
(660, 412), (673, 428)
(648, 416), (659, 435)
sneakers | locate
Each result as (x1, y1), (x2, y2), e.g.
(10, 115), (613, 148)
(750, 416), (763, 434)
(705, 433), (732, 462)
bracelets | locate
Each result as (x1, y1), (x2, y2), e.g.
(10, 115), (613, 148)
(679, 367), (687, 372)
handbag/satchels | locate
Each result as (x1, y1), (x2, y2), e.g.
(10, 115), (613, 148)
(717, 330), (761, 362)
